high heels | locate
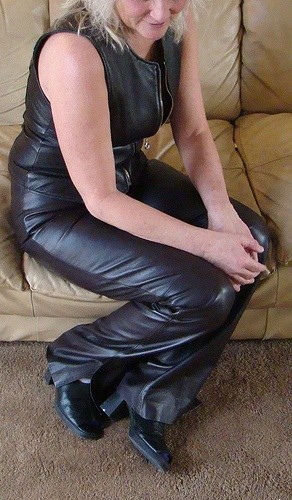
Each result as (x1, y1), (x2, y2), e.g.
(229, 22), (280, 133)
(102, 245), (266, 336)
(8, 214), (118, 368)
(99, 388), (172, 474)
(45, 364), (105, 440)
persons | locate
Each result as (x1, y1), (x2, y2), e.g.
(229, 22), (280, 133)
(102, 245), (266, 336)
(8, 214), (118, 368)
(9, 0), (269, 471)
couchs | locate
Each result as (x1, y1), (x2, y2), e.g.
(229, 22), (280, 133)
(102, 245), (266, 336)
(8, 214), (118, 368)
(0, 0), (292, 342)
